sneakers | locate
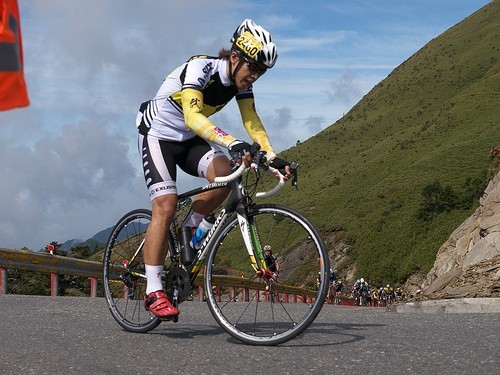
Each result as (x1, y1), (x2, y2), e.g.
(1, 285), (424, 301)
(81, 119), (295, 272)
(144, 290), (180, 317)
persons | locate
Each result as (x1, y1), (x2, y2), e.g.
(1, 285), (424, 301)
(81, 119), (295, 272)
(353, 278), (413, 304)
(263, 245), (279, 276)
(317, 268), (343, 296)
(137, 19), (294, 315)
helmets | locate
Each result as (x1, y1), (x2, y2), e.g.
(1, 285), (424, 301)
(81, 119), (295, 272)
(230, 18), (278, 69)
(264, 245), (272, 250)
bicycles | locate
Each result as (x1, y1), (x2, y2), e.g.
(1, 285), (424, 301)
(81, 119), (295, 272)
(264, 271), (415, 304)
(101, 142), (330, 346)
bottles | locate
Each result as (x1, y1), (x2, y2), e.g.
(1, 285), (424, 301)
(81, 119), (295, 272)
(188, 212), (217, 252)
(177, 226), (194, 264)
